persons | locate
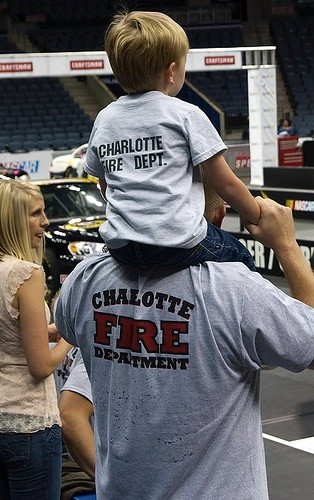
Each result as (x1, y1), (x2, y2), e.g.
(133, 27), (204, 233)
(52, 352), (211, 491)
(0, 177), (74, 500)
(48, 322), (96, 500)
(84, 10), (261, 275)
(54, 164), (314, 500)
(241, 116), (296, 141)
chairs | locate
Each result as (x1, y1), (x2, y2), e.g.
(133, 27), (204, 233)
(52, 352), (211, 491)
(0, 15), (314, 151)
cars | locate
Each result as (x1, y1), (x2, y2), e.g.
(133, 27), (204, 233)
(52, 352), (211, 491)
(28, 178), (109, 306)
(48, 143), (89, 177)
